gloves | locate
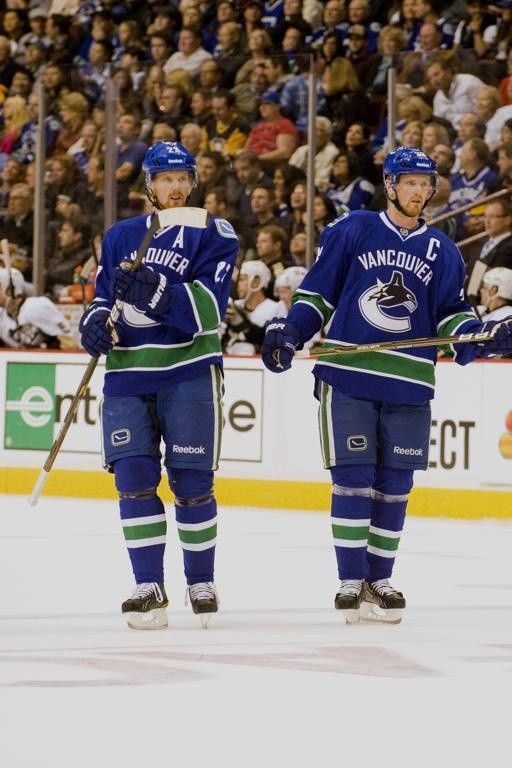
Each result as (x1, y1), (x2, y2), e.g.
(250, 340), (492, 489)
(452, 315), (511, 365)
(262, 317), (299, 374)
(77, 300), (126, 357)
(223, 298), (249, 332)
(111, 256), (175, 318)
(15, 326), (43, 345)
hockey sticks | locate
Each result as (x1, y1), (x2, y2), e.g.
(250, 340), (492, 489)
(26, 206), (214, 505)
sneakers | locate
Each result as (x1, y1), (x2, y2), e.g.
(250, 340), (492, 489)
(364, 569), (408, 610)
(188, 579), (219, 613)
(334, 578), (367, 610)
(120, 580), (169, 614)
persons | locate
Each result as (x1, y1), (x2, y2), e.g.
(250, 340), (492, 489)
(261, 144), (512, 614)
(79, 138), (238, 616)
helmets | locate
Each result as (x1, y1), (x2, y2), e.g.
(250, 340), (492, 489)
(382, 144), (440, 195)
(481, 266), (512, 301)
(141, 141), (200, 173)
(273, 266), (309, 298)
(238, 260), (271, 289)
(0, 267), (24, 306)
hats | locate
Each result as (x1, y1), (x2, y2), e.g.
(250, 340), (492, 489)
(254, 91), (281, 103)
